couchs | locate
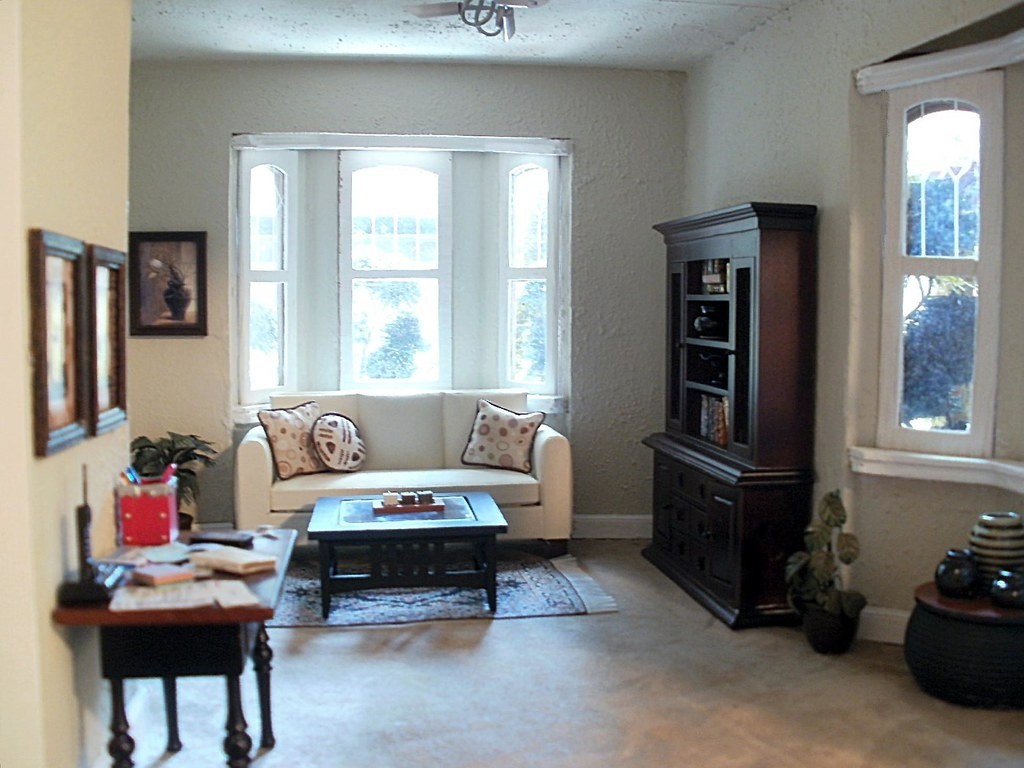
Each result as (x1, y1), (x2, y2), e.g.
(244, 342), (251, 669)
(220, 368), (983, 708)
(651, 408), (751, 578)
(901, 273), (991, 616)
(232, 389), (572, 562)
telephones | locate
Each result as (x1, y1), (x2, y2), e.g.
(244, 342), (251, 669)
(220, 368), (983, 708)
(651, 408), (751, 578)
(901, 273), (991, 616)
(57, 461), (126, 608)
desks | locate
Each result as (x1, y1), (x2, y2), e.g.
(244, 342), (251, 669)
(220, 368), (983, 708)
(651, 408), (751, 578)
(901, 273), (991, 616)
(54, 528), (299, 768)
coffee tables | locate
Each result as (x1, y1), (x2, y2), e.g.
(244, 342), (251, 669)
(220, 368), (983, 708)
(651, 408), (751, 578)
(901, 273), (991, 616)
(307, 491), (509, 620)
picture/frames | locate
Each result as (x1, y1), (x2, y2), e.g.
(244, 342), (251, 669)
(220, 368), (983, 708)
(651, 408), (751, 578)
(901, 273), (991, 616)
(89, 243), (130, 436)
(28, 228), (93, 456)
(127, 230), (209, 337)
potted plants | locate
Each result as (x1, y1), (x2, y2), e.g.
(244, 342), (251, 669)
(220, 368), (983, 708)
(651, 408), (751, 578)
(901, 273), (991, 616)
(129, 431), (222, 530)
(787, 490), (867, 654)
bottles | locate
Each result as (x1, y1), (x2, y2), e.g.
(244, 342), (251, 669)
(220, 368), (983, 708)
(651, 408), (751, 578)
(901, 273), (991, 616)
(694, 304), (727, 341)
(936, 512), (1024, 607)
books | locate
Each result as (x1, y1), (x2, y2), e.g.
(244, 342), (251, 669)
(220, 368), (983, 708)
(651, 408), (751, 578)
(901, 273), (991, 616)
(702, 259), (730, 293)
(134, 564), (193, 586)
(700, 394), (729, 445)
(191, 547), (276, 574)
(189, 531), (254, 547)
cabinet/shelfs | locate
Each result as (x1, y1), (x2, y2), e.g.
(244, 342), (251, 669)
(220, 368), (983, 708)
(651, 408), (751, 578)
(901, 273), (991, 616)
(642, 200), (819, 635)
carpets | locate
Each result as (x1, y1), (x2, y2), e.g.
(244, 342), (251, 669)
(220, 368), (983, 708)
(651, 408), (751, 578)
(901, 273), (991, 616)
(263, 543), (618, 629)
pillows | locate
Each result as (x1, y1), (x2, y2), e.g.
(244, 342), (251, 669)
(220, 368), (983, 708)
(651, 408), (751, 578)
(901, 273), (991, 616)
(257, 399), (328, 480)
(460, 400), (547, 474)
(312, 413), (368, 473)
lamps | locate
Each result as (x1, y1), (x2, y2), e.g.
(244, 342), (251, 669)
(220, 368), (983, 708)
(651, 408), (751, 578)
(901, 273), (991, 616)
(406, 0), (546, 41)
